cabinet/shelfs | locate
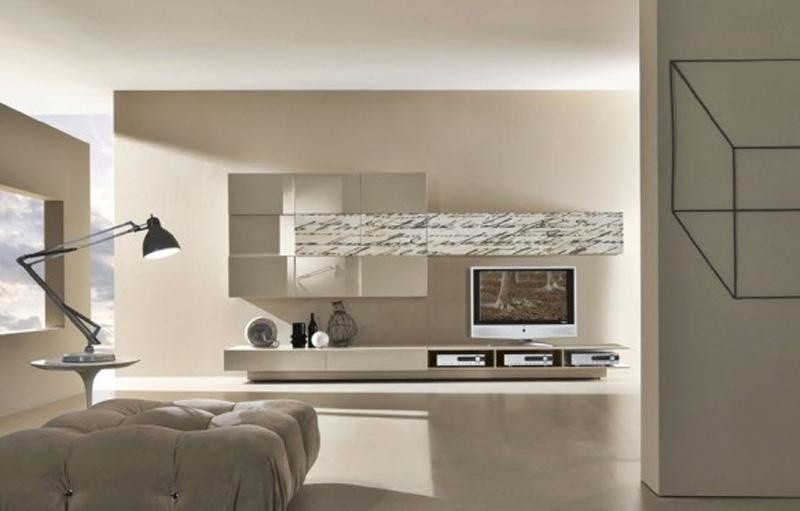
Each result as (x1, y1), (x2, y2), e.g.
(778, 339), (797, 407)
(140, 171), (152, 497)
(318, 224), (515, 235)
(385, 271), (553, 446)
(224, 169), (431, 306)
(222, 341), (631, 383)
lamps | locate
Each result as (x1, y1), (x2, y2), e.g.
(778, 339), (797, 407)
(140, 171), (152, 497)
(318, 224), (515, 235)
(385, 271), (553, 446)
(15, 212), (184, 364)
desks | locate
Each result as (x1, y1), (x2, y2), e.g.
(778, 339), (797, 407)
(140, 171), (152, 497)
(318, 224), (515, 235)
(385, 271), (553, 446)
(19, 352), (141, 411)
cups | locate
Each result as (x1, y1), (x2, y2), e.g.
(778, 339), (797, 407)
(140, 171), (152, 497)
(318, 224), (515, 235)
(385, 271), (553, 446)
(291, 322), (306, 348)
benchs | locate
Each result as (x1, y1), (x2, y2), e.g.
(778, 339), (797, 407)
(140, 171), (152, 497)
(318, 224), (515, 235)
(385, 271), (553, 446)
(0, 392), (321, 509)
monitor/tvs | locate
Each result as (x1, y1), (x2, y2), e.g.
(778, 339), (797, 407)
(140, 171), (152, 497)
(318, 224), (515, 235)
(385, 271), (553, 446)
(470, 266), (577, 349)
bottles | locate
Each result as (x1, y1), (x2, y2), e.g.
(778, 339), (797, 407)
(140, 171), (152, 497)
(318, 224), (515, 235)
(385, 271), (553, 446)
(308, 313), (319, 349)
